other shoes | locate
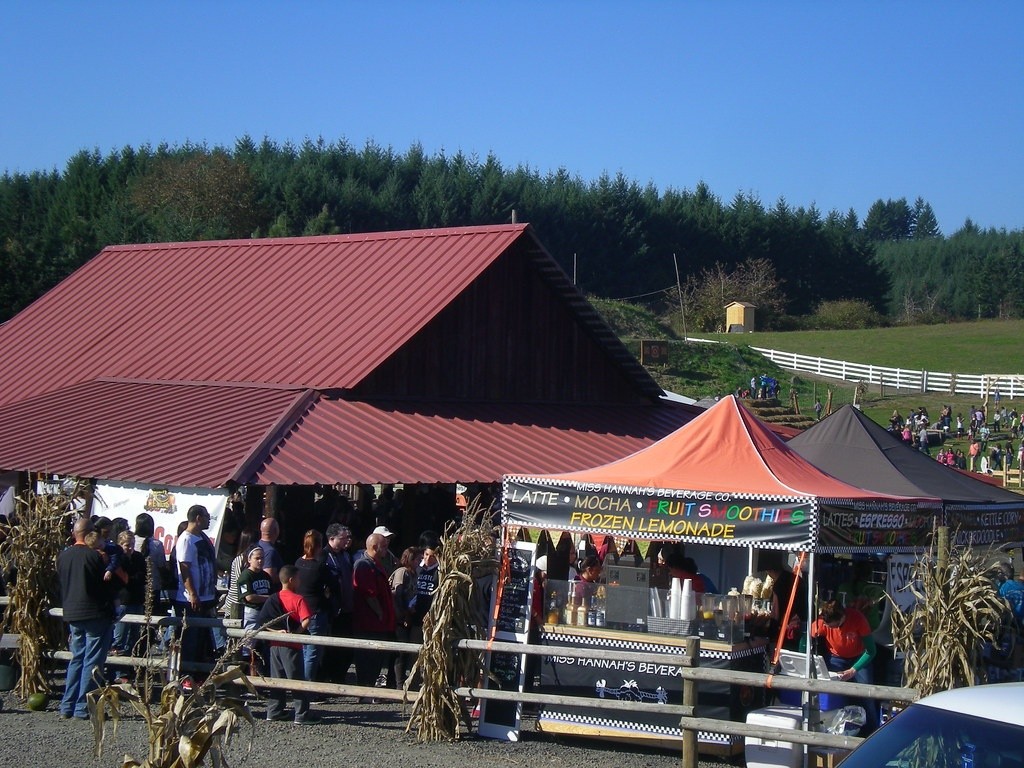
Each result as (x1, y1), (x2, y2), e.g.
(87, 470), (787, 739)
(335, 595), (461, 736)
(107, 645), (126, 657)
(309, 695), (328, 704)
(179, 678), (206, 692)
(265, 711), (290, 721)
(355, 696), (382, 704)
(60, 711), (108, 721)
(111, 604), (128, 625)
(294, 715), (323, 725)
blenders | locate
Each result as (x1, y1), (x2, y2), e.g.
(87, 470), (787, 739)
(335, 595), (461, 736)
(697, 592), (717, 639)
(716, 595), (738, 641)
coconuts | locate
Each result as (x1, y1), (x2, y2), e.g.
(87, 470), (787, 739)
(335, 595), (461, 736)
(28, 693), (50, 710)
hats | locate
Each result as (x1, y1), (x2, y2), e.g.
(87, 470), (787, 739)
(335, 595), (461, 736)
(372, 526), (394, 537)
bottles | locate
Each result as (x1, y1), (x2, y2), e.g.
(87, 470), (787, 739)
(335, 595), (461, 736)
(222, 571), (228, 588)
(727, 587), (740, 596)
(546, 590), (606, 628)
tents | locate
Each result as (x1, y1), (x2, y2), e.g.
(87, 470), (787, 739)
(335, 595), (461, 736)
(489, 393), (944, 744)
(783, 403), (1024, 615)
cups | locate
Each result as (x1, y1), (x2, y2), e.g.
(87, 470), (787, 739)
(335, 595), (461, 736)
(647, 577), (696, 621)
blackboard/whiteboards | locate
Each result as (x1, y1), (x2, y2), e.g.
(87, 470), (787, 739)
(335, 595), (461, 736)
(478, 539), (537, 742)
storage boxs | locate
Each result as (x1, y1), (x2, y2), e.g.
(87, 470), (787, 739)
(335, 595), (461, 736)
(775, 648), (846, 708)
(808, 745), (852, 768)
(746, 705), (820, 768)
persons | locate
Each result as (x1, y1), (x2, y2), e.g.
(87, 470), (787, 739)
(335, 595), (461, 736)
(531, 538), (600, 628)
(0, 481), (505, 725)
(814, 398), (822, 423)
(994, 385), (1000, 410)
(835, 559), (887, 645)
(666, 554), (719, 635)
(800, 602), (876, 735)
(959, 562), (1024, 685)
(713, 373), (781, 400)
(887, 405), (1024, 478)
